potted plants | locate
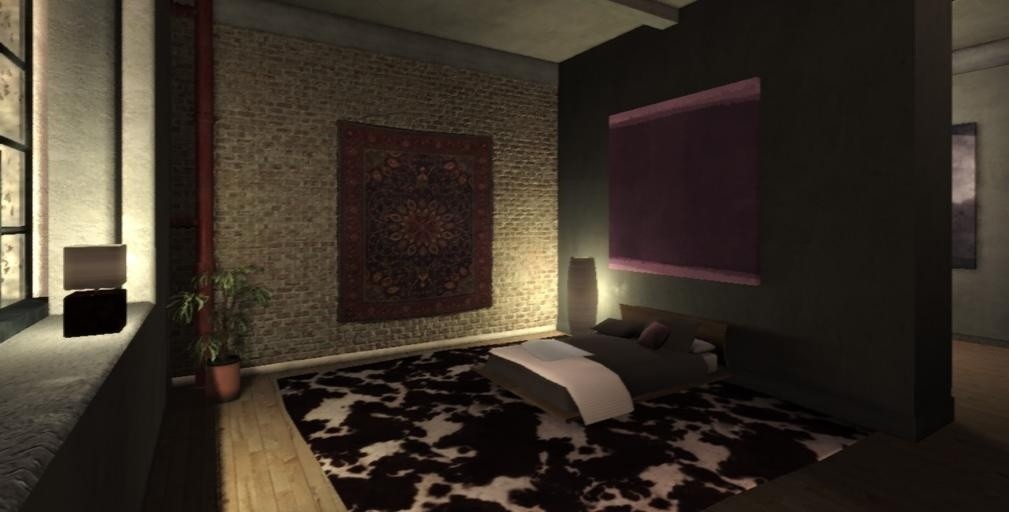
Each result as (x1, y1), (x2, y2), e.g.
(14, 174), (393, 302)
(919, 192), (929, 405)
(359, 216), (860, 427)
(164, 262), (274, 406)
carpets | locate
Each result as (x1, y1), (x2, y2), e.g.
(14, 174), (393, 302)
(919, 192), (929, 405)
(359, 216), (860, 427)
(271, 339), (872, 512)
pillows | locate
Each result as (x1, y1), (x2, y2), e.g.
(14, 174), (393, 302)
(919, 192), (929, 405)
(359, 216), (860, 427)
(590, 318), (670, 348)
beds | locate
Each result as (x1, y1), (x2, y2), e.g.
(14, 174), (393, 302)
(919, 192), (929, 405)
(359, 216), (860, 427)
(476, 303), (729, 423)
